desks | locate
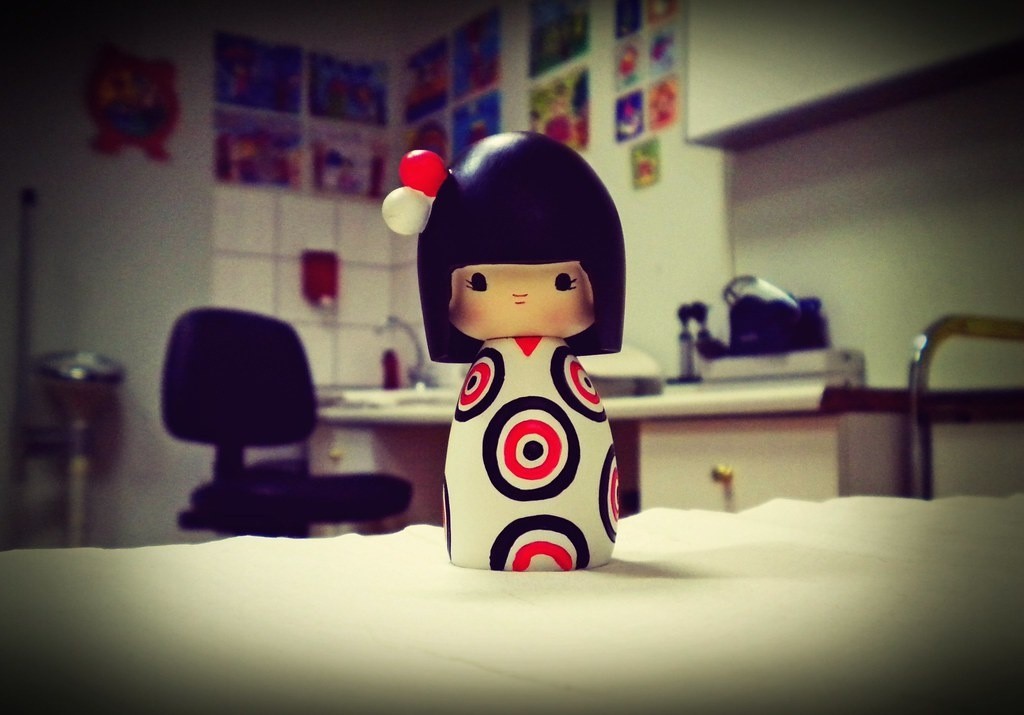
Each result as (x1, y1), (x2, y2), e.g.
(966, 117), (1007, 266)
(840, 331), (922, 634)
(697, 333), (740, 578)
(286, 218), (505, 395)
(299, 370), (1017, 535)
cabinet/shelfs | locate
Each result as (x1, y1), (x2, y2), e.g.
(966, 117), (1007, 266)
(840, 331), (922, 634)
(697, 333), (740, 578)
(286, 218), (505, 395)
(684, 1), (1024, 153)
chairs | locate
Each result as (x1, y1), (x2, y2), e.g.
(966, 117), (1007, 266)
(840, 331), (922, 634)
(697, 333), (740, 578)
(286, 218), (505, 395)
(159, 304), (417, 540)
